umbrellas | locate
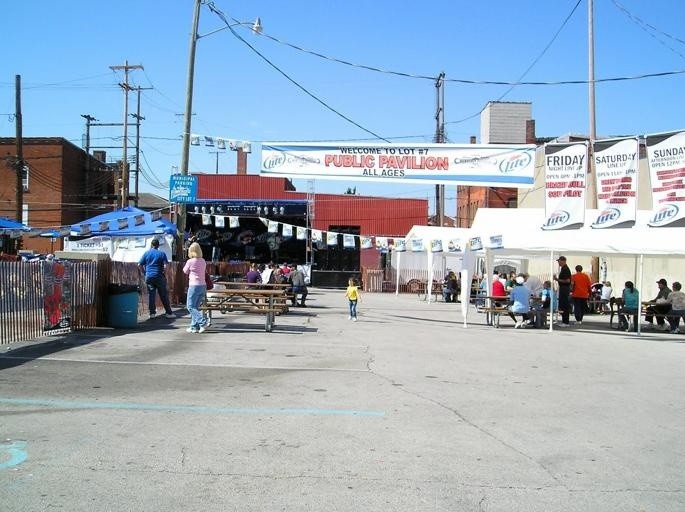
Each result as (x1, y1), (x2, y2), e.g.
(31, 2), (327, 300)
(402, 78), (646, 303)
(591, 283), (603, 290)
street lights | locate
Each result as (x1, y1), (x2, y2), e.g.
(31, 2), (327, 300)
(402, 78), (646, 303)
(178, 2), (266, 313)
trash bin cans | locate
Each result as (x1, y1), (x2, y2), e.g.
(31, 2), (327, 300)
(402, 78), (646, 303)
(105, 282), (141, 330)
(227, 271), (244, 282)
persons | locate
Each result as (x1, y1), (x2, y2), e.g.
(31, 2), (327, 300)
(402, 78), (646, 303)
(476, 271), (543, 316)
(644, 279), (674, 330)
(534, 280), (558, 328)
(245, 260), (309, 308)
(243, 230), (255, 258)
(552, 256), (572, 328)
(268, 232), (282, 263)
(619, 281), (640, 332)
(45, 253), (54, 261)
(571, 265), (592, 325)
(599, 281), (613, 315)
(588, 287), (601, 314)
(209, 229), (224, 263)
(183, 242), (207, 334)
(344, 273), (362, 322)
(443, 271), (458, 303)
(508, 276), (531, 328)
(137, 238), (177, 319)
(660, 282), (685, 334)
(187, 235), (200, 258)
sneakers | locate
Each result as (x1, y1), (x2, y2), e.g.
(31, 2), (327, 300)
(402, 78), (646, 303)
(166, 313), (177, 319)
(348, 316), (357, 322)
(644, 323), (680, 333)
(186, 323), (211, 333)
(515, 322), (526, 329)
(559, 321), (581, 327)
(150, 314), (155, 318)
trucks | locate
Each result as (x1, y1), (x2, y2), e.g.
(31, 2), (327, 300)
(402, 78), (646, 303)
(387, 248), (489, 296)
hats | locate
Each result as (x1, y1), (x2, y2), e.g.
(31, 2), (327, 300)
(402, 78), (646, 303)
(656, 278), (667, 283)
(515, 276), (525, 284)
(288, 264), (297, 268)
(556, 256), (566, 262)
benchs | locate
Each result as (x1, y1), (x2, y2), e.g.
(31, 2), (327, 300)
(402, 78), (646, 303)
(195, 282), (296, 334)
(469, 296), (685, 331)
